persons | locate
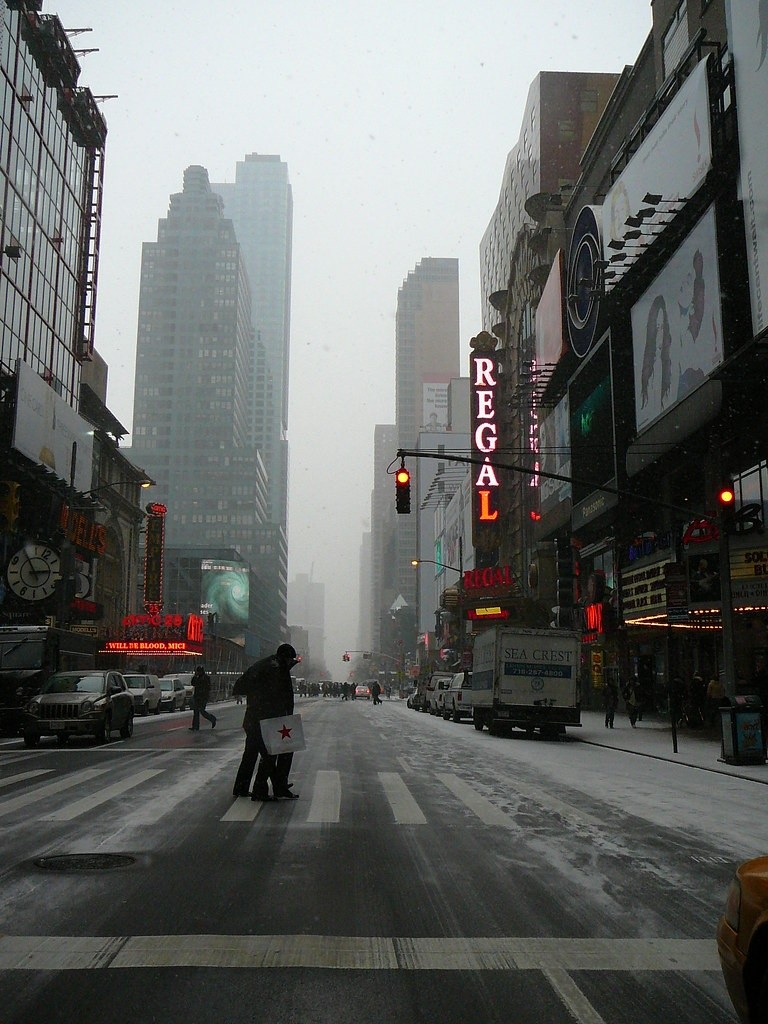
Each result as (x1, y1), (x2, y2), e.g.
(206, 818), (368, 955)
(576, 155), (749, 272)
(690, 559), (720, 601)
(664, 672), (688, 730)
(708, 672), (726, 741)
(298, 681), (359, 701)
(371, 681), (382, 705)
(384, 683), (391, 700)
(232, 644), (300, 800)
(426, 414), (442, 427)
(622, 674), (643, 729)
(188, 665), (217, 732)
(685, 671), (707, 728)
(602, 674), (619, 729)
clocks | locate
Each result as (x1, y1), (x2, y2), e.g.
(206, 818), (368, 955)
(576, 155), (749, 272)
(3, 538), (63, 607)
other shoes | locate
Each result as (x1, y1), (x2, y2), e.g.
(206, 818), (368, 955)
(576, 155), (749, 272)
(188, 727), (199, 731)
(251, 794), (274, 801)
(212, 721), (216, 728)
(233, 791), (252, 797)
(274, 790), (300, 799)
(288, 783), (294, 789)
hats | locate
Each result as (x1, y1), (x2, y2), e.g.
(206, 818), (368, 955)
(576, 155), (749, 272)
(277, 644), (296, 658)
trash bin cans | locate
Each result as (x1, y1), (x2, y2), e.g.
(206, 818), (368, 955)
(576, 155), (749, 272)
(717, 696), (768, 766)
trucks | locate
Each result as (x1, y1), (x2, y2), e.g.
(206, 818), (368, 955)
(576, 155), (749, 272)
(463, 624), (588, 737)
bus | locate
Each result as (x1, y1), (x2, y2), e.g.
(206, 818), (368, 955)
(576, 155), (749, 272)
(0, 624), (96, 737)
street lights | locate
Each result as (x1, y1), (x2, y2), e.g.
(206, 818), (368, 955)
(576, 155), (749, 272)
(59, 441), (155, 627)
(410, 535), (465, 670)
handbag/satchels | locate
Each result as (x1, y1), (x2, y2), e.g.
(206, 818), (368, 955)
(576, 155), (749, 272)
(260, 711), (306, 755)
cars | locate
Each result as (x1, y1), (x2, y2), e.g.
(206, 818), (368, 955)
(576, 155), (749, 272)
(157, 678), (186, 713)
(407, 669), (457, 718)
(354, 685), (371, 699)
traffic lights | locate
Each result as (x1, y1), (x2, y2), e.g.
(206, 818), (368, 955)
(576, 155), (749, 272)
(347, 654), (350, 661)
(395, 468), (411, 514)
(342, 655), (345, 661)
(298, 655), (302, 663)
(721, 484), (736, 516)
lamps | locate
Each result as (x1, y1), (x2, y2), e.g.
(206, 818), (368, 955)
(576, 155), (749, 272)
(508, 360), (557, 409)
(568, 192), (691, 302)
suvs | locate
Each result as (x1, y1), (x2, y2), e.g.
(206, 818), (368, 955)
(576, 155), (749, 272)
(161, 673), (196, 709)
(20, 668), (135, 748)
(438, 671), (473, 723)
(119, 673), (162, 716)
